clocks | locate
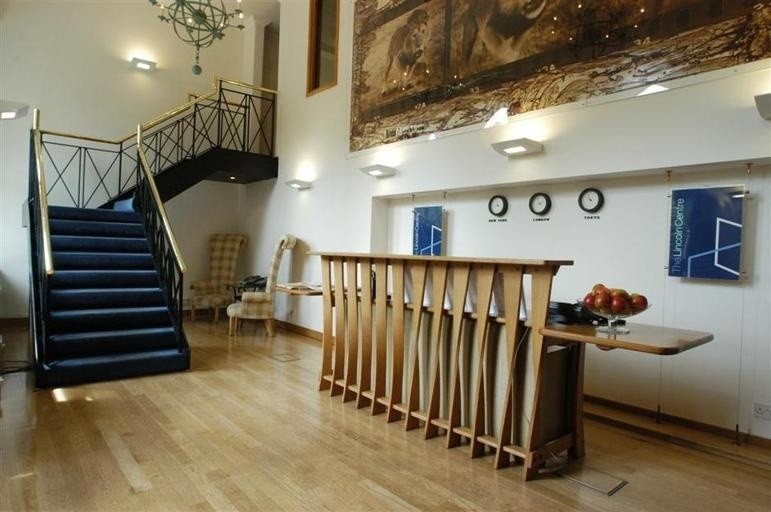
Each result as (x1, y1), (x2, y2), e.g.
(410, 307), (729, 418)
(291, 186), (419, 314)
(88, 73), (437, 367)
(487, 187), (604, 217)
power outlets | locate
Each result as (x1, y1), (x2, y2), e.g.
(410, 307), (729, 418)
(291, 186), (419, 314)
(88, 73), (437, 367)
(751, 401), (770, 420)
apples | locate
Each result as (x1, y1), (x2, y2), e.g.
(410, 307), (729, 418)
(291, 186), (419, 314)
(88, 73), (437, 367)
(585, 283), (647, 314)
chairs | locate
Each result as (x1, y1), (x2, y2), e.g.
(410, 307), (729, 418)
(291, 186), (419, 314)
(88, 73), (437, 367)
(186, 232), (296, 338)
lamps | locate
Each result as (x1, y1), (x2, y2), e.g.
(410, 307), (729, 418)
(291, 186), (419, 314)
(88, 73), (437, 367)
(489, 137), (544, 159)
(284, 178), (310, 191)
(128, 56), (156, 73)
(147, 0), (246, 75)
(357, 164), (395, 179)
(0, 103), (28, 121)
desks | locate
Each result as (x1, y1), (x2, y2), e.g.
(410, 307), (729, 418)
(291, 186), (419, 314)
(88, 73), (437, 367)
(274, 250), (713, 482)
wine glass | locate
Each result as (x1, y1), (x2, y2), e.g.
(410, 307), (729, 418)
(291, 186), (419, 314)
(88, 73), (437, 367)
(577, 297), (654, 336)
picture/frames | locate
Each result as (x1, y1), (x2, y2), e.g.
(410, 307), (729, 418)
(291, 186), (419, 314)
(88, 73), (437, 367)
(343, 0), (771, 161)
